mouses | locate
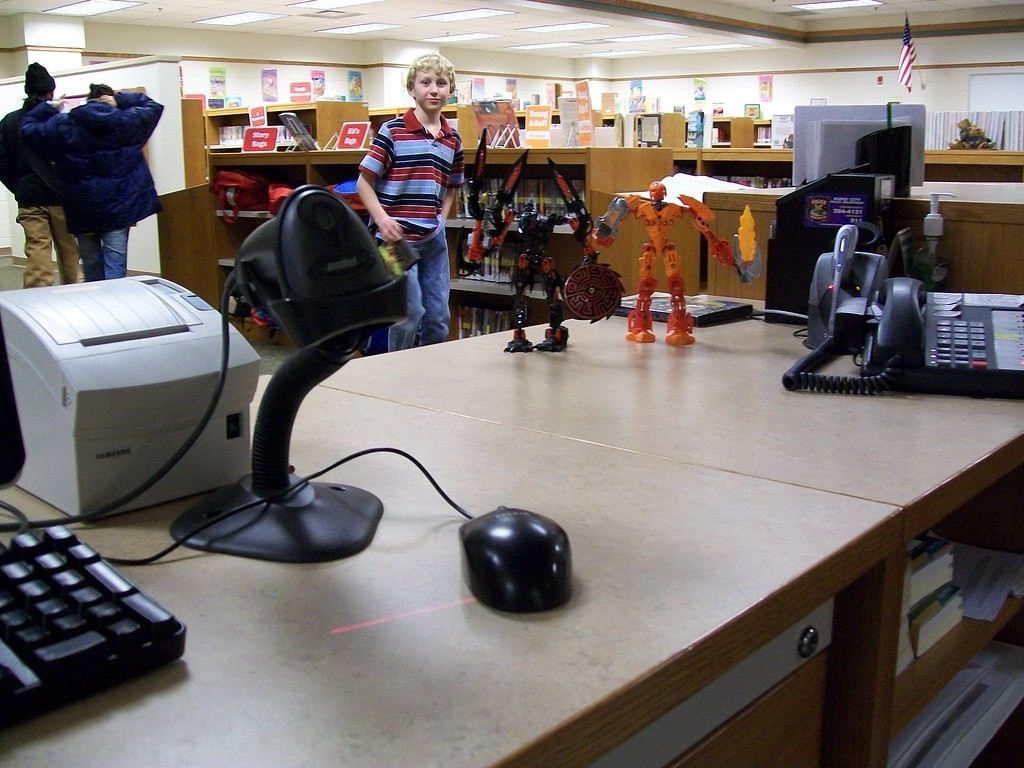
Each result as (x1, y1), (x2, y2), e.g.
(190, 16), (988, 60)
(457, 506), (572, 615)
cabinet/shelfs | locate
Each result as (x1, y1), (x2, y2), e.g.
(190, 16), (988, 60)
(0, 100), (1024, 768)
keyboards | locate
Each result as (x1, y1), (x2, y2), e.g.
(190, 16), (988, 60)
(0, 524), (186, 729)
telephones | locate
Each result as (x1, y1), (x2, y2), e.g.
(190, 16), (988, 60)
(860, 277), (1024, 400)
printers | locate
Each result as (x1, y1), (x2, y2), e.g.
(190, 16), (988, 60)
(0, 275), (261, 518)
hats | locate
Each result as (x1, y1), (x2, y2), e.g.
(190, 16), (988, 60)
(24, 62), (56, 94)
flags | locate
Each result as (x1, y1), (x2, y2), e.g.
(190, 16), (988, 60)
(898, 17), (916, 94)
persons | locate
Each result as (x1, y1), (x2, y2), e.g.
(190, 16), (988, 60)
(468, 129), (627, 353)
(19, 83), (164, 282)
(593, 181), (761, 345)
(0, 62), (81, 287)
(356, 53), (465, 353)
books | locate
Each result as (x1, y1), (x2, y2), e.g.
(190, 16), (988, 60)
(925, 111), (1024, 151)
(455, 178), (584, 339)
(219, 124), (312, 145)
(757, 127), (772, 143)
(714, 174), (791, 188)
(895, 535), (964, 675)
